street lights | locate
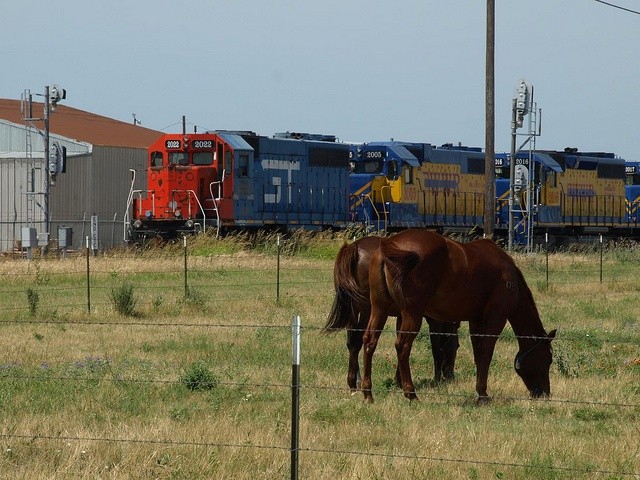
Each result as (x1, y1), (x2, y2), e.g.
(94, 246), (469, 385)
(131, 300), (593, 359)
(50, 84), (65, 103)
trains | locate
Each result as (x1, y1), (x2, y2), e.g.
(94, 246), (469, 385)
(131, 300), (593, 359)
(123, 130), (640, 247)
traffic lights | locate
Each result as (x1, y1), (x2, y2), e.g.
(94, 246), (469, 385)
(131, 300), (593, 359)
(51, 142), (66, 175)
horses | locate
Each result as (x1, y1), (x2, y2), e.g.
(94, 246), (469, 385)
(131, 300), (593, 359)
(362, 228), (558, 405)
(320, 236), (461, 396)
